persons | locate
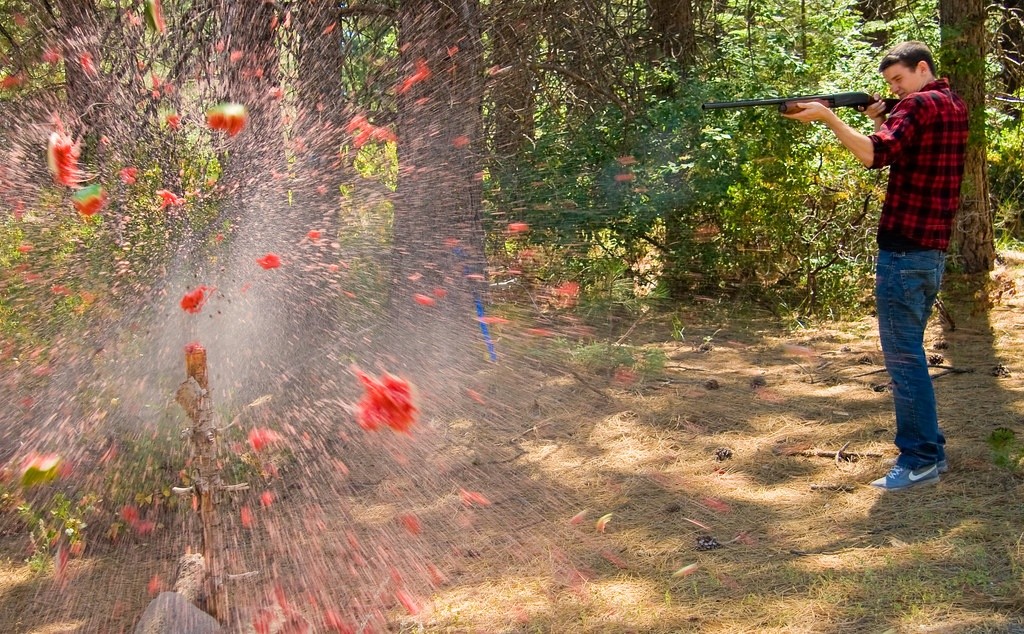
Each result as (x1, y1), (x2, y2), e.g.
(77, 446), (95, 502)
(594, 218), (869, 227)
(778, 37), (969, 493)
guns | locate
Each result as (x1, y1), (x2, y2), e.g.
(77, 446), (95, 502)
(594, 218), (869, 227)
(701, 88), (902, 117)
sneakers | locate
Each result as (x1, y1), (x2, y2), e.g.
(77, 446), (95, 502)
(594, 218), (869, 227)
(871, 465), (940, 491)
(937, 459), (948, 474)
